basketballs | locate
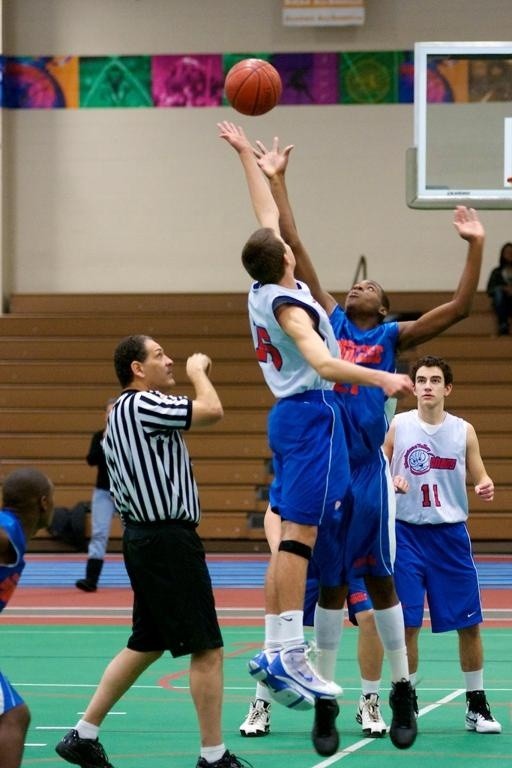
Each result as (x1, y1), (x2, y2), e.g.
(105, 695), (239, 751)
(224, 58), (283, 117)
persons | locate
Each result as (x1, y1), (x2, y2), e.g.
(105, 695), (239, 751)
(216, 118), (415, 710)
(381, 354), (503, 733)
(239, 501), (389, 738)
(487, 243), (511, 338)
(250, 136), (487, 755)
(56, 333), (251, 768)
(75, 396), (117, 591)
(0, 468), (56, 767)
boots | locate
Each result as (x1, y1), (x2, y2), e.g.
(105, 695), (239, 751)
(76, 559), (103, 592)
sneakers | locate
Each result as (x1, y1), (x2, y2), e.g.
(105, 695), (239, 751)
(56, 729), (114, 768)
(465, 706), (501, 733)
(356, 694), (386, 738)
(196, 750), (244, 768)
(240, 699), (271, 737)
(248, 647), (315, 711)
(266, 644), (337, 700)
(313, 697), (338, 756)
(389, 678), (418, 749)
